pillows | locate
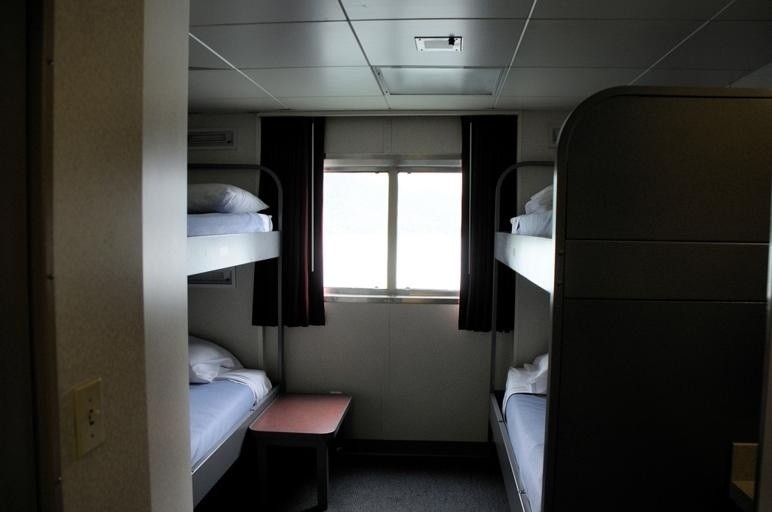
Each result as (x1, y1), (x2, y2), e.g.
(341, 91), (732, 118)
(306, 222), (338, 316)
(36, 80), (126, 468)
(525, 184), (552, 213)
(531, 352), (548, 394)
(188, 183), (272, 215)
(187, 336), (244, 384)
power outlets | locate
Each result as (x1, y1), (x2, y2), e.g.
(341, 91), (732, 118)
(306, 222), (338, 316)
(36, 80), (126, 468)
(73, 377), (111, 459)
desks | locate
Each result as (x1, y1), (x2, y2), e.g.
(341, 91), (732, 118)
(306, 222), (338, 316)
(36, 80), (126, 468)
(248, 392), (353, 510)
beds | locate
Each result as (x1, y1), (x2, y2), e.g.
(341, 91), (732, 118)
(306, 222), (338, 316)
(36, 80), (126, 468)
(488, 85), (772, 512)
(188, 163), (286, 509)
